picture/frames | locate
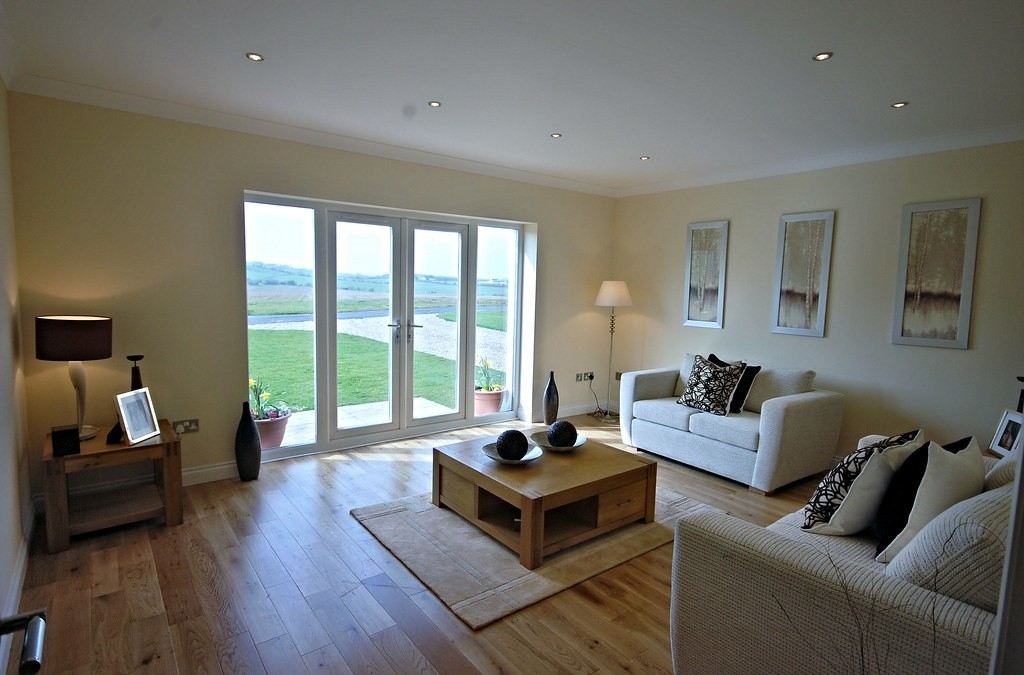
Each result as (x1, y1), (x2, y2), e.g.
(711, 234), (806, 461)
(989, 408), (1024, 458)
(890, 198), (982, 349)
(113, 387), (161, 445)
(682, 221), (728, 329)
(771, 210), (835, 337)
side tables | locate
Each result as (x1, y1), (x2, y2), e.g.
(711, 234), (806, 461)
(42, 418), (184, 553)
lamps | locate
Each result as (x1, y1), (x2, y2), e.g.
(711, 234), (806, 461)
(592, 280), (634, 424)
(34, 314), (113, 441)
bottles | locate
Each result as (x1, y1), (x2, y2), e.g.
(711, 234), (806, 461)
(232, 401), (261, 482)
(542, 369), (560, 425)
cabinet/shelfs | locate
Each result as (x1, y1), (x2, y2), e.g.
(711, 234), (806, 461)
(432, 427), (658, 570)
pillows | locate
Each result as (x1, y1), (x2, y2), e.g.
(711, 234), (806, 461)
(800, 428), (925, 536)
(676, 354), (747, 418)
(706, 352), (762, 414)
(874, 434), (986, 562)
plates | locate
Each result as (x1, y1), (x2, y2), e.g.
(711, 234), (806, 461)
(529, 430), (587, 452)
(481, 440), (542, 464)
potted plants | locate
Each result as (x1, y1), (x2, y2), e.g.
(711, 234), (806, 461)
(474, 355), (507, 415)
(248, 374), (292, 449)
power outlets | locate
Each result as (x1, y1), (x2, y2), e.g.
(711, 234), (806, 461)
(575, 372), (583, 382)
(173, 420), (187, 435)
(583, 371), (593, 380)
(187, 418), (199, 432)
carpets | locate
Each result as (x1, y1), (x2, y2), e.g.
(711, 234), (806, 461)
(349, 486), (732, 629)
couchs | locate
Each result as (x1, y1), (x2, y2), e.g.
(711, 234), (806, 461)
(670, 434), (1024, 675)
(620, 352), (846, 496)
